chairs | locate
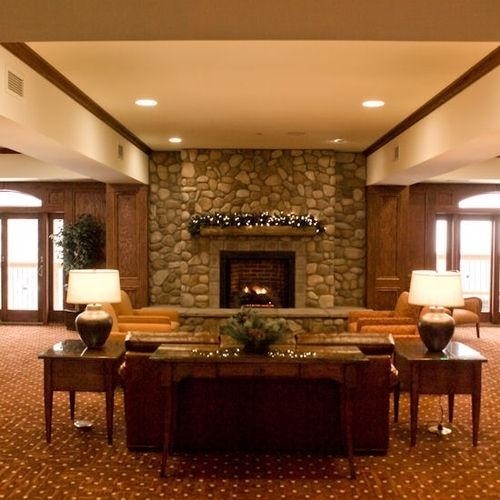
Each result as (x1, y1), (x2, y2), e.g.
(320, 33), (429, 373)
(102, 303), (171, 340)
(112, 289), (180, 333)
(343, 292), (422, 333)
(361, 306), (452, 339)
(452, 298), (482, 337)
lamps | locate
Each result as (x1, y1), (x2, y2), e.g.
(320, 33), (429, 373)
(408, 271), (465, 353)
(66, 269), (121, 348)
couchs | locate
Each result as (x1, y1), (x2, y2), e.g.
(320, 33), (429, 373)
(126, 333), (398, 457)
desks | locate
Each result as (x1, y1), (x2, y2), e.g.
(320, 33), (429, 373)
(147, 344), (370, 480)
(37, 340), (125, 444)
(395, 342), (487, 447)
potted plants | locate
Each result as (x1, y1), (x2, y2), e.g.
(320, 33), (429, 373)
(221, 306), (290, 354)
(49, 212), (106, 330)
